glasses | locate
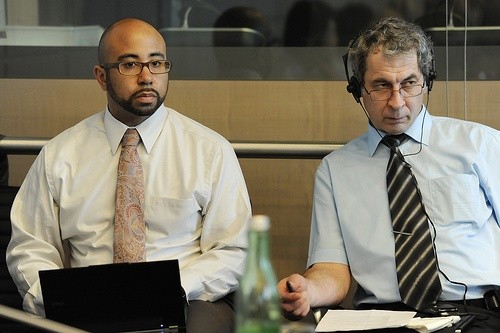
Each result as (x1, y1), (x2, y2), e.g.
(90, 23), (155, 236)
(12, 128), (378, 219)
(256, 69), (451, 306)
(100, 60), (172, 76)
(362, 74), (427, 101)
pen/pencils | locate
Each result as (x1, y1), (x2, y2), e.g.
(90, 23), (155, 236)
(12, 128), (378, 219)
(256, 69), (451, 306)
(286, 281), (294, 293)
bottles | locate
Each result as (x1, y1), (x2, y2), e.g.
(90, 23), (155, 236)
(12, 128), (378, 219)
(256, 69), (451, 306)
(234, 215), (283, 333)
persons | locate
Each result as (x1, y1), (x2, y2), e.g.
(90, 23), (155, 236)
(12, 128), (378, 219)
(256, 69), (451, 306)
(276, 17), (500, 320)
(6, 18), (252, 333)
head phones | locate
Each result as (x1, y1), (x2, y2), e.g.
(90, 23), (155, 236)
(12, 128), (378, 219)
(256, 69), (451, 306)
(342, 36), (437, 103)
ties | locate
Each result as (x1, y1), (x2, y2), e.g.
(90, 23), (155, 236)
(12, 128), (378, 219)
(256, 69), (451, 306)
(113, 129), (147, 263)
(380, 133), (444, 312)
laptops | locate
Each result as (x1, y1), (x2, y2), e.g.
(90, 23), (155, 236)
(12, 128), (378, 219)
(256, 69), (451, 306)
(39, 259), (185, 333)
